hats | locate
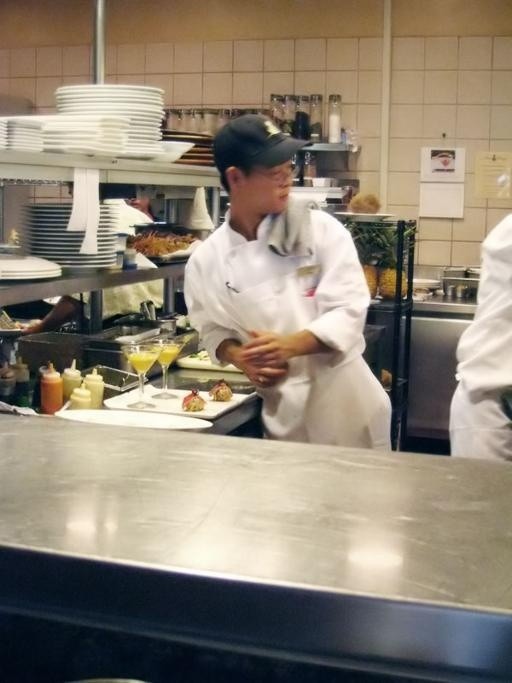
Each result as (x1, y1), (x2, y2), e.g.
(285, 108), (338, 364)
(213, 113), (314, 176)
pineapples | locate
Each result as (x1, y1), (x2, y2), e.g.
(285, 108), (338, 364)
(355, 227), (378, 297)
(378, 230), (413, 298)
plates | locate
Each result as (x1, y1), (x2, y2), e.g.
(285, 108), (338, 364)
(2, 114), (129, 158)
(55, 410), (213, 433)
(407, 279), (441, 289)
(0, 119), (7, 150)
(54, 85), (166, 159)
(6, 118), (46, 153)
(0, 254), (60, 281)
(104, 384), (258, 420)
(334, 210), (391, 222)
(15, 202), (118, 274)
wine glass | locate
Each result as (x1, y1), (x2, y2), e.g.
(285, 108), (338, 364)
(152, 342), (185, 399)
(120, 344), (160, 409)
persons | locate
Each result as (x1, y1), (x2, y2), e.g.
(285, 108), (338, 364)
(183, 112), (394, 452)
(447, 207), (512, 464)
(16, 180), (167, 334)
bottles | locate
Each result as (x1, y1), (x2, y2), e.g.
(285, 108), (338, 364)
(303, 151), (317, 187)
(32, 361), (54, 412)
(0, 366), (15, 404)
(271, 91), (285, 135)
(295, 94), (307, 140)
(327, 94), (341, 143)
(14, 357), (29, 406)
(63, 358), (84, 399)
(41, 363), (62, 413)
(83, 367), (105, 409)
(162, 108), (269, 137)
(284, 94), (293, 135)
(308, 93), (322, 141)
(70, 384), (92, 410)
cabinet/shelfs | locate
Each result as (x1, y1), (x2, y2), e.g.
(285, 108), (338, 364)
(285, 140), (360, 207)
(1, 147), (222, 332)
(343, 219), (416, 452)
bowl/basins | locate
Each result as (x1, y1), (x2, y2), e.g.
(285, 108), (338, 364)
(156, 140), (195, 163)
(311, 177), (332, 188)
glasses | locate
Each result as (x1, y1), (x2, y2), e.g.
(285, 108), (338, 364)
(248, 162), (296, 179)
